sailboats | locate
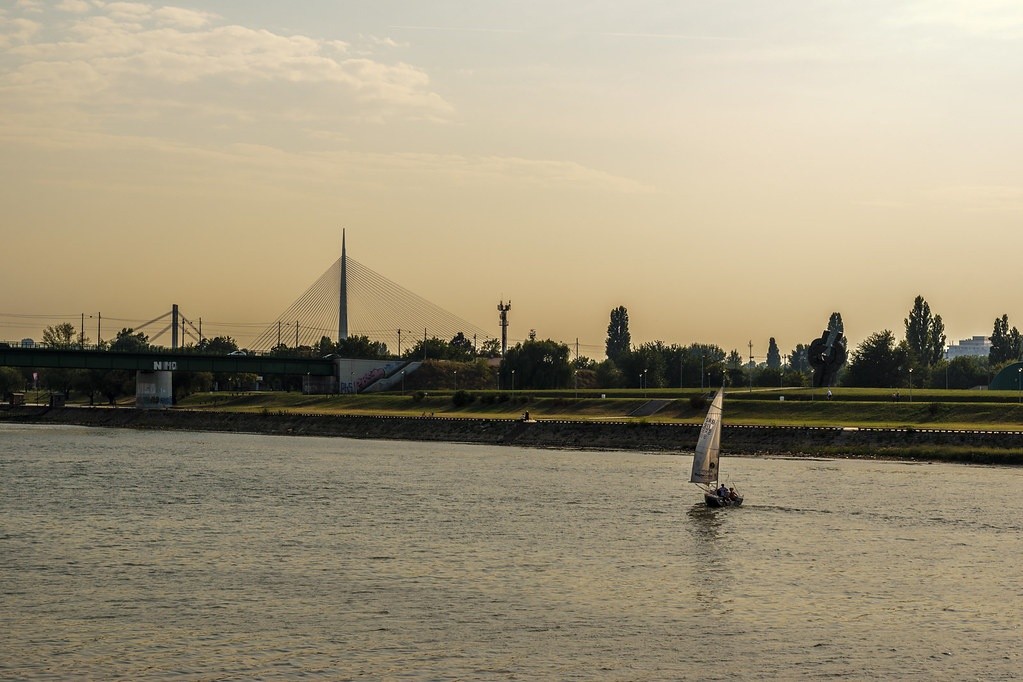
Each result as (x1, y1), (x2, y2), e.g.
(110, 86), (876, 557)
(689, 387), (744, 507)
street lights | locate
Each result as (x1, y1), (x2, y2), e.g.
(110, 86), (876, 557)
(702, 355), (705, 387)
(308, 372), (310, 394)
(908, 368), (914, 402)
(402, 370), (405, 395)
(351, 371), (355, 393)
(811, 369), (815, 400)
(1017, 368), (1023, 402)
(511, 370), (515, 396)
(454, 371), (456, 390)
(780, 373), (783, 387)
(497, 372), (500, 390)
(723, 370), (726, 379)
(574, 371), (578, 398)
(639, 374), (643, 389)
(708, 372), (710, 387)
(644, 369), (648, 398)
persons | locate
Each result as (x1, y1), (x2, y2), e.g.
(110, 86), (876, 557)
(719, 483), (727, 496)
(525, 411), (529, 421)
(728, 488), (737, 495)
(826, 389), (832, 400)
(896, 392), (900, 402)
(522, 414), (526, 420)
(892, 391), (896, 402)
